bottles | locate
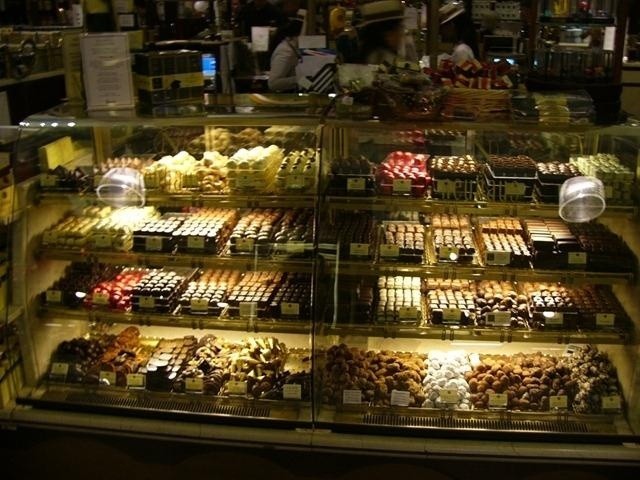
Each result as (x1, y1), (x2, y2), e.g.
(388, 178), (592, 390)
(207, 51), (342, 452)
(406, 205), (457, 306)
(576, 0), (590, 17)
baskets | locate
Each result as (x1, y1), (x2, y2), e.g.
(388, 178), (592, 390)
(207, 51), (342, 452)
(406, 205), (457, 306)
(440, 86), (510, 120)
(377, 44), (441, 121)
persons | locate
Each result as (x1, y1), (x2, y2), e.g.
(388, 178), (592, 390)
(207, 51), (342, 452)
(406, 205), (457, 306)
(437, 0), (481, 70)
(351, 1), (413, 67)
(268, 12), (311, 93)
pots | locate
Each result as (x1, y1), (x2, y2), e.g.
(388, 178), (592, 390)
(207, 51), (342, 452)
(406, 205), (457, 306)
(559, 24), (584, 44)
(542, 0), (572, 17)
